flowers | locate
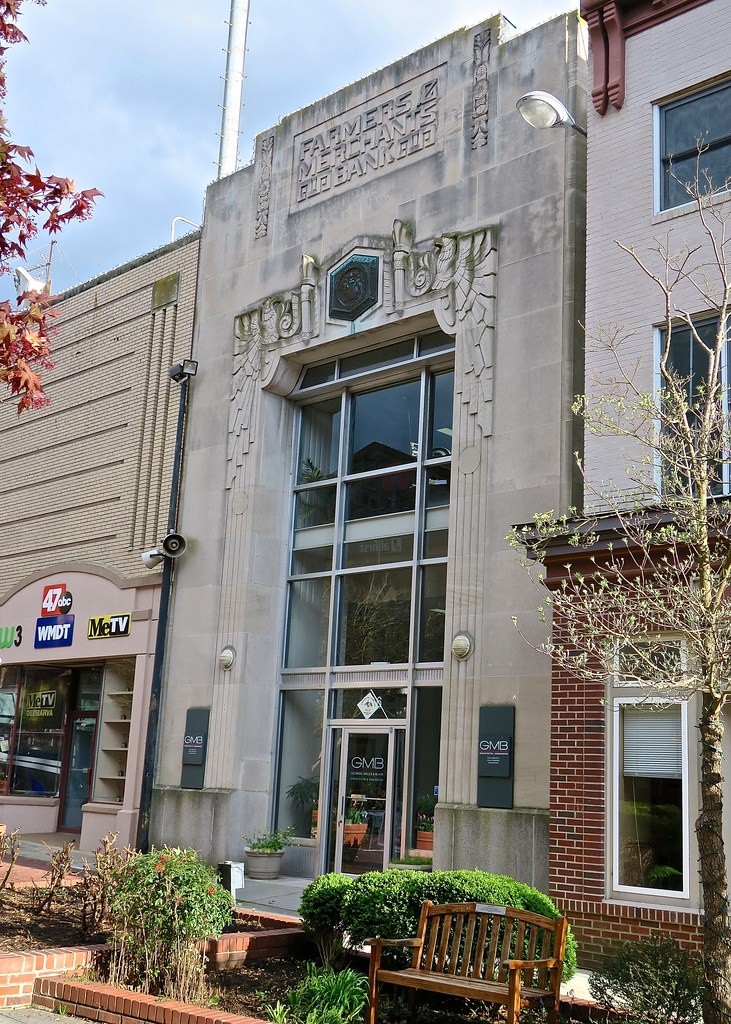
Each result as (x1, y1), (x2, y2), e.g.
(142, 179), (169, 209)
(239, 825), (301, 855)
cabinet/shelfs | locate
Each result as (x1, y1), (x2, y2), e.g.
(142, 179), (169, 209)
(92, 664), (133, 804)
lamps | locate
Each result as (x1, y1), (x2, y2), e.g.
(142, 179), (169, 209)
(451, 630), (475, 662)
(219, 645), (237, 671)
(515, 90), (587, 138)
(168, 358), (199, 386)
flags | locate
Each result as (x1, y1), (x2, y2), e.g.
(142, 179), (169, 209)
(357, 693), (380, 719)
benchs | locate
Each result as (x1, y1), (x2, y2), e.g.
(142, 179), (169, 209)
(363, 899), (569, 1024)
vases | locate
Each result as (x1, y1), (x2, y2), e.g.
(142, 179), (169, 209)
(243, 846), (286, 880)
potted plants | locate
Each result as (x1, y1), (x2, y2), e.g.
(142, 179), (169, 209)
(312, 802), (318, 822)
(331, 807), (368, 846)
(414, 792), (437, 850)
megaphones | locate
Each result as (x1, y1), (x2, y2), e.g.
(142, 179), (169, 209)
(163, 533), (187, 558)
(141, 548), (165, 569)
